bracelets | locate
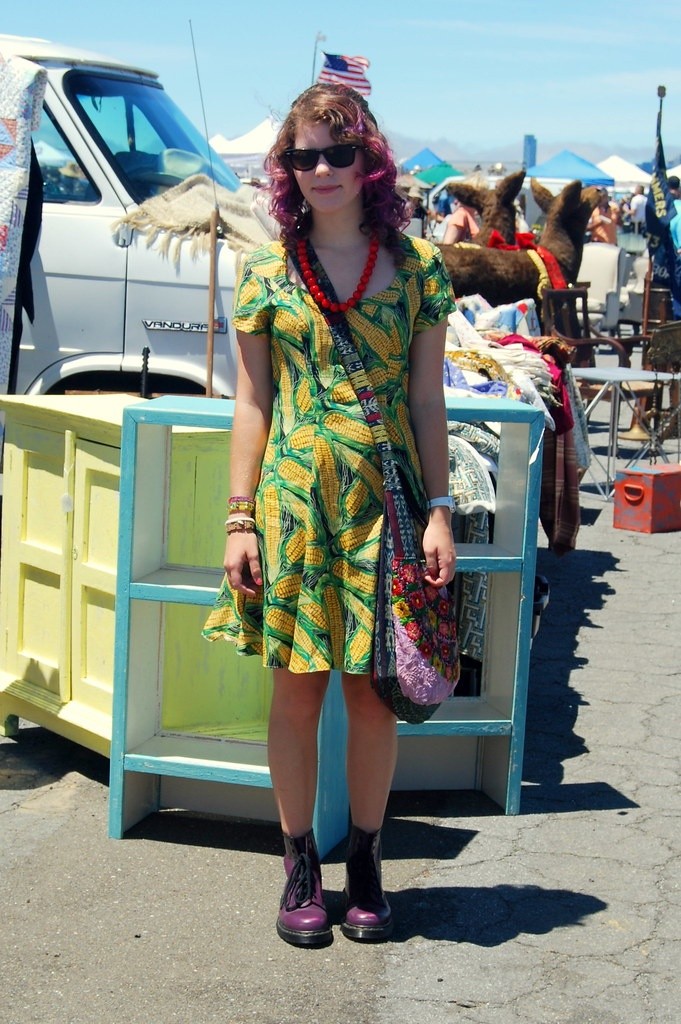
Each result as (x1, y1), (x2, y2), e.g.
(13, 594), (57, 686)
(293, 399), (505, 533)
(224, 496), (256, 532)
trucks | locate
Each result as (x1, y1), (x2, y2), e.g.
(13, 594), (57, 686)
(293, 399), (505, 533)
(0, 30), (481, 398)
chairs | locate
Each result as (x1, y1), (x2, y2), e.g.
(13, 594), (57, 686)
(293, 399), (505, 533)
(541, 284), (662, 439)
(574, 243), (651, 353)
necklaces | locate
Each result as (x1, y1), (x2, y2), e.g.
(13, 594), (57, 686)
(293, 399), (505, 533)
(297, 235), (378, 312)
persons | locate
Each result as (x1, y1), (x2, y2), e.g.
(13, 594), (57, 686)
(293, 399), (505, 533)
(623, 176), (681, 234)
(438, 174), (486, 246)
(402, 185), (427, 220)
(587, 187), (620, 246)
(200, 80), (461, 944)
(668, 177), (681, 321)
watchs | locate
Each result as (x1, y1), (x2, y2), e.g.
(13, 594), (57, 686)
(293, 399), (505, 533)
(427, 496), (456, 513)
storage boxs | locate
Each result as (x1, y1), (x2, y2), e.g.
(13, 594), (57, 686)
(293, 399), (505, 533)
(613, 463), (681, 535)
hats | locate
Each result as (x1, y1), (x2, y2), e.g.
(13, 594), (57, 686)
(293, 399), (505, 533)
(406, 185), (424, 202)
(595, 186), (608, 193)
(58, 161), (82, 177)
(136, 146), (206, 187)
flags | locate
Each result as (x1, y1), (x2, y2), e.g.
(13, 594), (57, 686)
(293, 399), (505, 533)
(315, 54), (371, 95)
(644, 136), (678, 256)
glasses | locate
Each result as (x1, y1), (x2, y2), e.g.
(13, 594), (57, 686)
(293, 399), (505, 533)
(281, 142), (364, 172)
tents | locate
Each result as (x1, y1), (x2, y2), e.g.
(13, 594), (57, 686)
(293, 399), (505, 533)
(207, 114), (681, 184)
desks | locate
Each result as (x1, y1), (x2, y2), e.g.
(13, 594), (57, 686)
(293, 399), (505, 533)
(572, 365), (680, 502)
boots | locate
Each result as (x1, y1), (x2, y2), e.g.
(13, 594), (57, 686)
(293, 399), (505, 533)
(276, 827), (332, 944)
(341, 825), (393, 940)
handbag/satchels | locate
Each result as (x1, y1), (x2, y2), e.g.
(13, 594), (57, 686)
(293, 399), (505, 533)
(371, 455), (460, 726)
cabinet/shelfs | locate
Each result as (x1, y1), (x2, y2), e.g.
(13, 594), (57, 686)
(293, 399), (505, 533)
(0, 390), (547, 842)
